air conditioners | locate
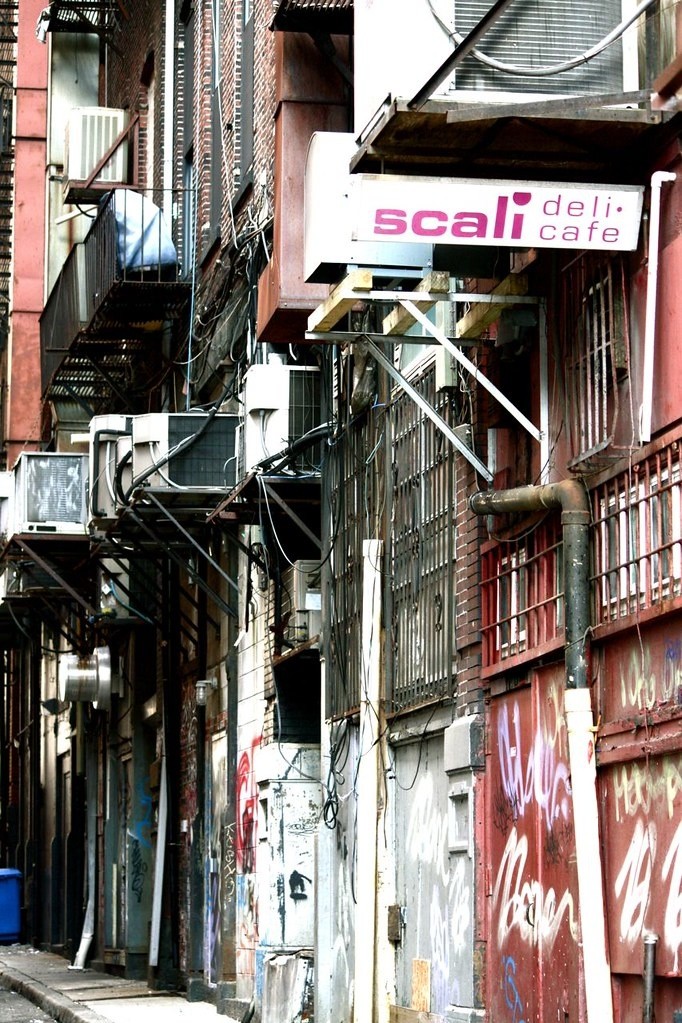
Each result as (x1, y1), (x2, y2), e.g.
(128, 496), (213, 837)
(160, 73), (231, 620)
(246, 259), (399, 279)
(130, 407), (241, 492)
(62, 105), (129, 192)
(84, 413), (136, 529)
(301, 128), (516, 289)
(234, 363), (324, 486)
(5, 451), (92, 544)
(352, 0), (639, 146)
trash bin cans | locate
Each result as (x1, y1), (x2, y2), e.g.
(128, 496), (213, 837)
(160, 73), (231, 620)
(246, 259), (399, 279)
(0, 868), (21, 945)
